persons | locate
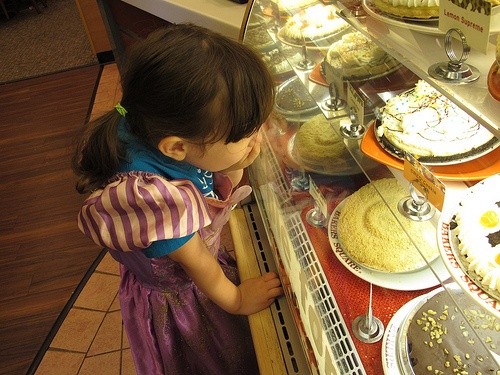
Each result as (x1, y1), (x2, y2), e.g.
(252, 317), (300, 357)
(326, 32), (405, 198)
(72, 20), (285, 375)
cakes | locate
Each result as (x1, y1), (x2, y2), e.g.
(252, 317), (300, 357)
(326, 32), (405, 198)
(276, 1), (500, 375)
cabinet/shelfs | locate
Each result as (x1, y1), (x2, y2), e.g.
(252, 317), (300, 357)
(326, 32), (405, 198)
(239, 0), (500, 375)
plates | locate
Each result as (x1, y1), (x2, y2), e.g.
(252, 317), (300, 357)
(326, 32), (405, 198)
(436, 172), (500, 322)
(328, 195), (453, 291)
(273, 75), (318, 115)
(285, 24), (352, 44)
(373, 87), (500, 166)
(381, 293), (427, 375)
(361, 0), (500, 36)
(319, 57), (403, 83)
(287, 132), (378, 175)
